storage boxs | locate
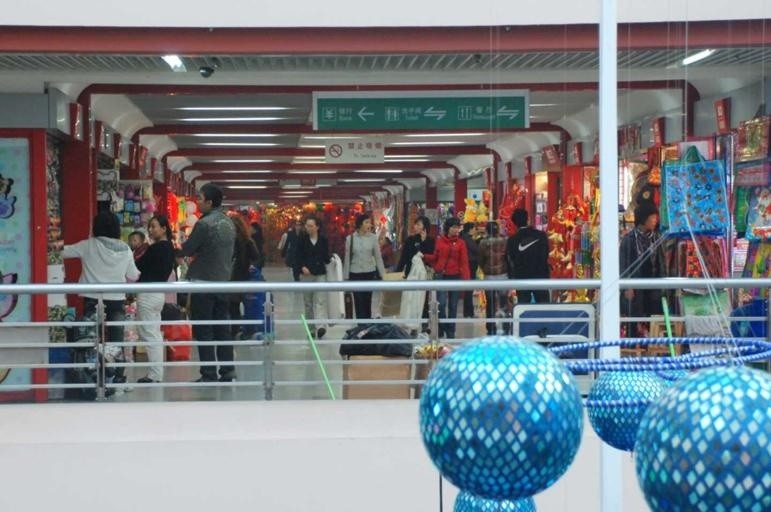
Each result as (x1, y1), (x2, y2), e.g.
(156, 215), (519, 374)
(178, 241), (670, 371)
(341, 354), (415, 399)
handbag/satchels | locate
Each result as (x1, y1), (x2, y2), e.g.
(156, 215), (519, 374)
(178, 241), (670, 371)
(344, 292), (354, 320)
(433, 272), (444, 280)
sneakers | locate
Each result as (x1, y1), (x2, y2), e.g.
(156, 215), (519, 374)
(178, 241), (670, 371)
(307, 326), (327, 340)
(134, 375), (242, 384)
(409, 327), (456, 339)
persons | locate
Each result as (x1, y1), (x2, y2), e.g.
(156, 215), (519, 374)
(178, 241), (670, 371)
(228, 212), (261, 340)
(621, 200), (669, 349)
(172, 241), (189, 308)
(276, 209), (552, 335)
(59, 209), (142, 397)
(127, 227), (149, 258)
(248, 222), (265, 271)
(182, 183), (238, 382)
(134, 212), (176, 382)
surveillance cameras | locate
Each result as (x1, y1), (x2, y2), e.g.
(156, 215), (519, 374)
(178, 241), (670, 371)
(199, 66), (213, 77)
